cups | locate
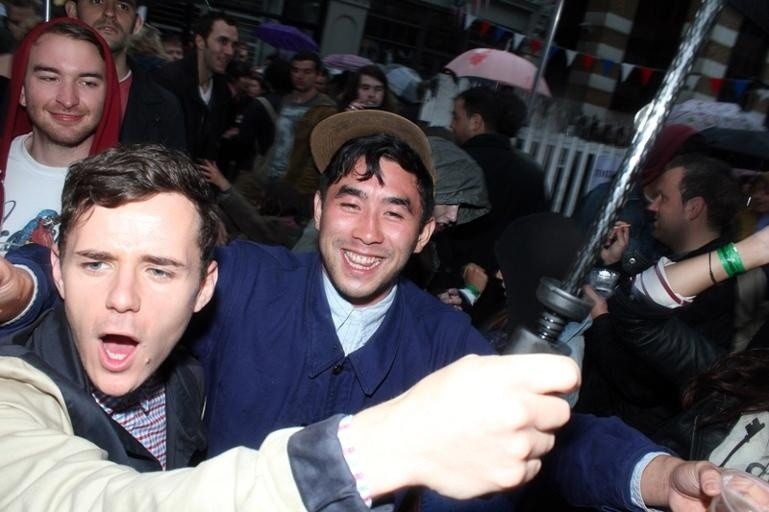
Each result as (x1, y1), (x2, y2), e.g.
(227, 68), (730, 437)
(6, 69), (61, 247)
(707, 467), (769, 512)
(589, 268), (621, 297)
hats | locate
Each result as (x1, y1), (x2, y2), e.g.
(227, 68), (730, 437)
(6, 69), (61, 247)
(310, 109), (437, 197)
